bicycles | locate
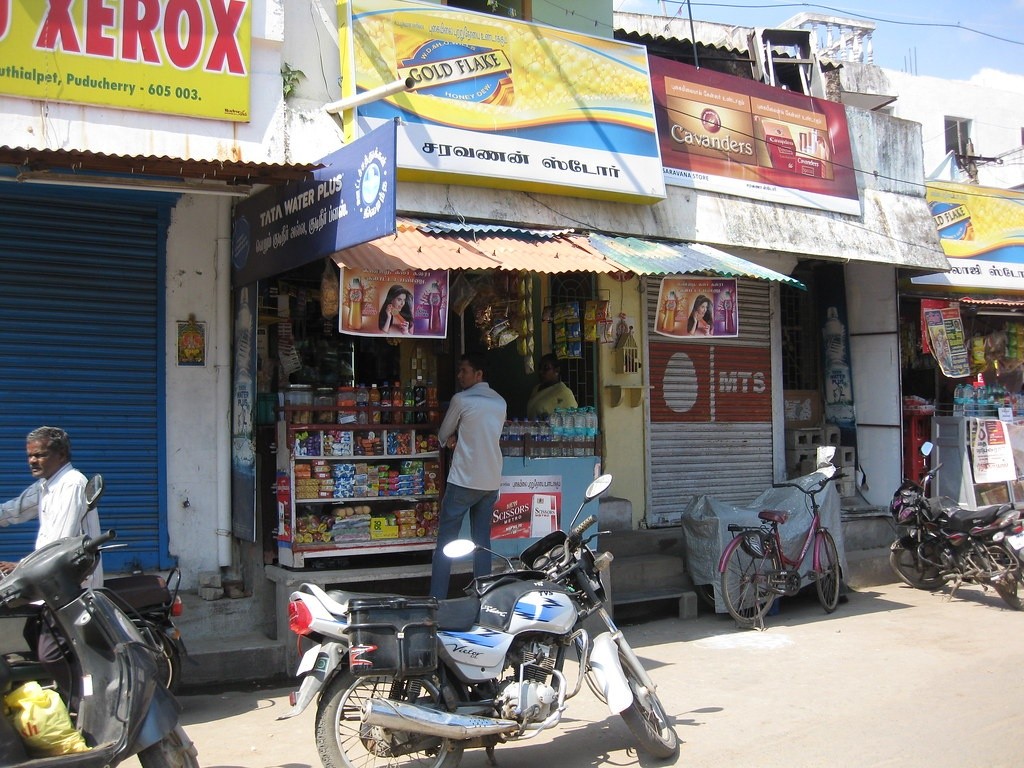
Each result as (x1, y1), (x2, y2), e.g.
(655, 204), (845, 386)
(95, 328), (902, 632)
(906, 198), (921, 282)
(718, 466), (850, 631)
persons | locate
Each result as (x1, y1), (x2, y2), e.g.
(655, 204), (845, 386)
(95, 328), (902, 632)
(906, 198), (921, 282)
(686, 294), (714, 336)
(526, 353), (578, 421)
(377, 284), (414, 335)
(0, 425), (104, 697)
(429, 354), (507, 599)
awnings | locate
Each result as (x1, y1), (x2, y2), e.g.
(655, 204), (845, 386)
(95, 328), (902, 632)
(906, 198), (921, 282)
(330, 217), (809, 293)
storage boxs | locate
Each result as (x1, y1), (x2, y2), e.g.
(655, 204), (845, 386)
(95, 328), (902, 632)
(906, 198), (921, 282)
(902, 412), (932, 495)
(783, 389), (821, 429)
(760, 120), (797, 172)
(794, 131), (828, 179)
(345, 595), (439, 675)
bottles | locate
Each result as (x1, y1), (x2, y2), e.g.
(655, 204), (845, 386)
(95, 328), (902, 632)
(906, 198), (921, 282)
(499, 405), (598, 458)
(283, 375), (440, 426)
(822, 307), (853, 422)
(724, 291), (733, 333)
(663, 291), (677, 332)
(429, 282), (440, 334)
(953, 383), (1024, 416)
(349, 278), (363, 331)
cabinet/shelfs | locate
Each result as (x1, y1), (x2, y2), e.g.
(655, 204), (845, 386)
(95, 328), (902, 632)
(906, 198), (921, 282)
(273, 406), (449, 570)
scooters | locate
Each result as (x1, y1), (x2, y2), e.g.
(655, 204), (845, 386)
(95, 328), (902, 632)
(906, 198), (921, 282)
(0, 474), (201, 768)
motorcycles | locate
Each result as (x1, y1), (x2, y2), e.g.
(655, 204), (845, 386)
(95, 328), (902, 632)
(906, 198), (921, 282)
(0, 559), (200, 711)
(274, 474), (678, 768)
(889, 442), (1024, 611)
